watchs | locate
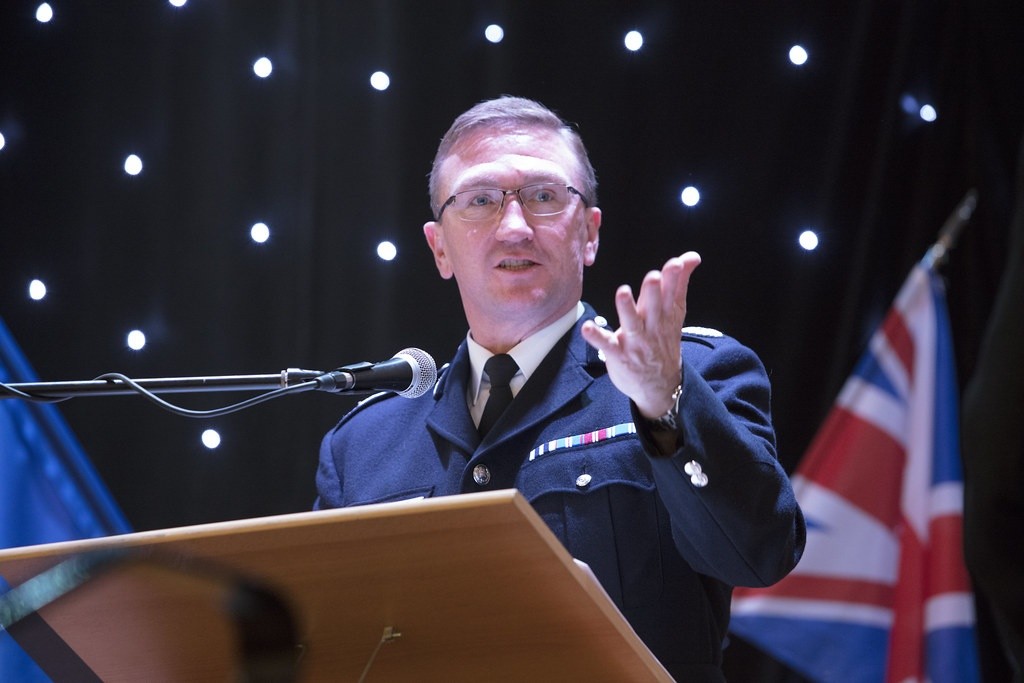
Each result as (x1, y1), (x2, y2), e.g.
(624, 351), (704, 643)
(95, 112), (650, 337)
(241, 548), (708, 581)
(639, 386), (682, 431)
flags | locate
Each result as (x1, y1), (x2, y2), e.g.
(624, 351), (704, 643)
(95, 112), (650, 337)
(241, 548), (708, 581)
(725, 254), (977, 683)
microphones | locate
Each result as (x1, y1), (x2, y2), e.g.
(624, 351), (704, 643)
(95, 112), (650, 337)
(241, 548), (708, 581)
(313, 347), (437, 398)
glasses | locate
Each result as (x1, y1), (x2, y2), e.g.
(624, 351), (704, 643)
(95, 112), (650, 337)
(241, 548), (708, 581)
(438, 183), (589, 222)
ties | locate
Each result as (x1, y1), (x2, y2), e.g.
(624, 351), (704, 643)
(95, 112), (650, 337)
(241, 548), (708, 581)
(477, 354), (520, 441)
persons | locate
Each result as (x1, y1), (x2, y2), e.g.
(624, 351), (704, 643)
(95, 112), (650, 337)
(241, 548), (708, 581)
(311, 96), (808, 683)
